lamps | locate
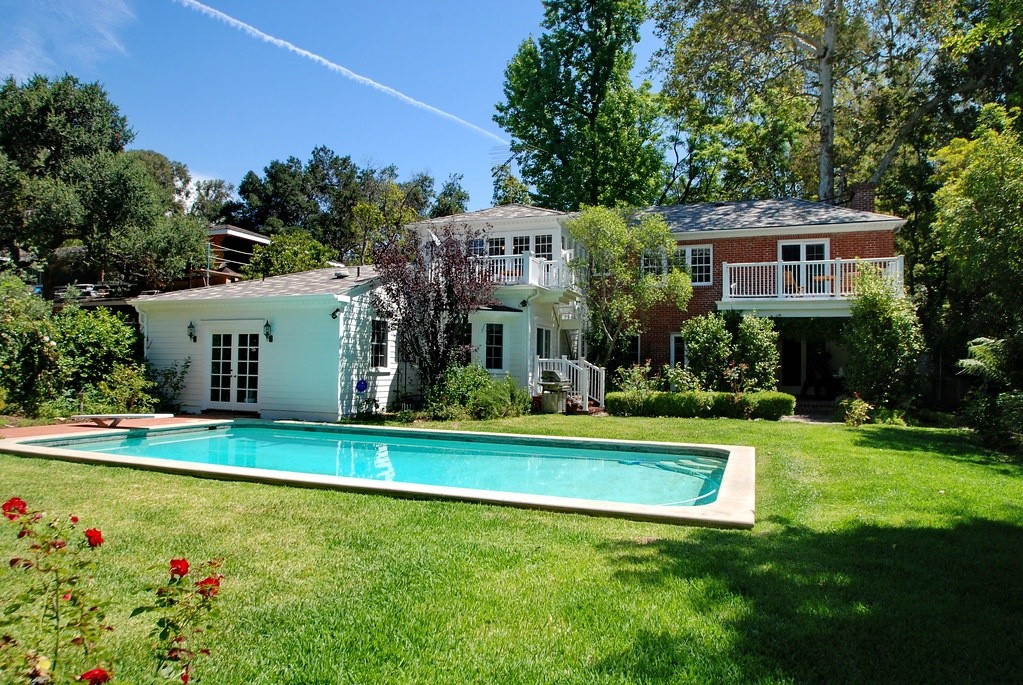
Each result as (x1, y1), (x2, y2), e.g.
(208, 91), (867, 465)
(186, 320), (197, 342)
(263, 320), (273, 342)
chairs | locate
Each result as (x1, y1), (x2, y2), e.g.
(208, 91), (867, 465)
(785, 271), (803, 293)
(841, 272), (856, 291)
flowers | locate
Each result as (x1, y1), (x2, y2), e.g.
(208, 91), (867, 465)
(43, 335), (57, 349)
(0, 495), (227, 685)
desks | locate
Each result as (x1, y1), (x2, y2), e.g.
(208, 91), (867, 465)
(813, 275), (835, 293)
